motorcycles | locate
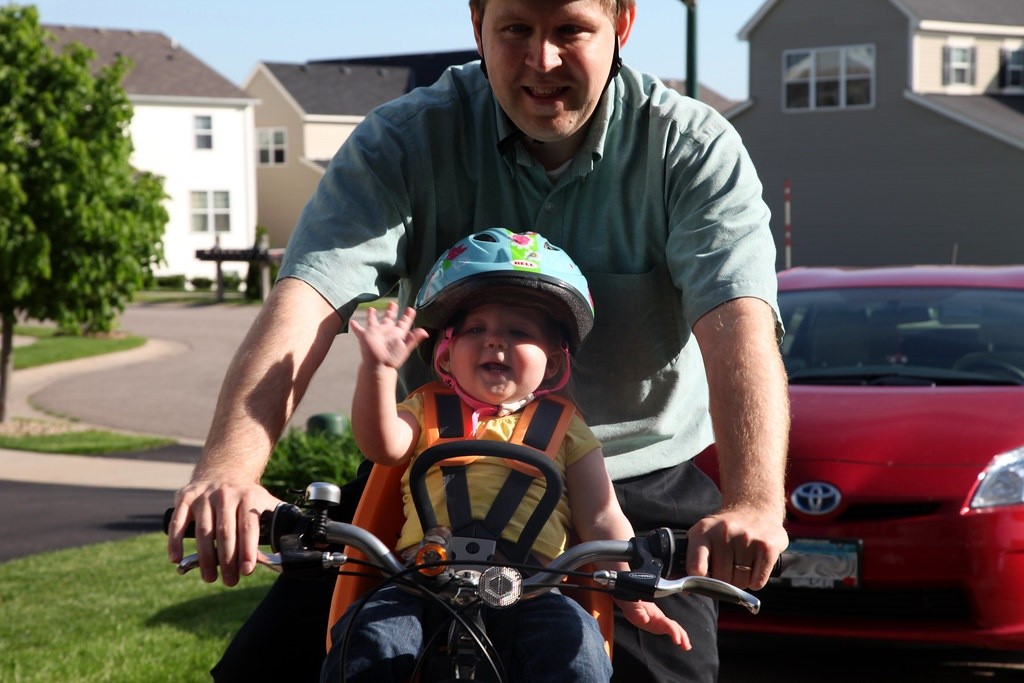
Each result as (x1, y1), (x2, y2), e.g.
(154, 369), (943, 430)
(161, 480), (785, 683)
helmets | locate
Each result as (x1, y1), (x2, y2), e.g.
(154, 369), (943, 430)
(413, 225), (596, 367)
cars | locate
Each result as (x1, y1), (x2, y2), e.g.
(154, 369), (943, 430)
(691, 262), (1023, 683)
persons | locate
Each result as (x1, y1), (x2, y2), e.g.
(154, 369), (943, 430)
(322, 226), (692, 683)
(165, 0), (789, 682)
